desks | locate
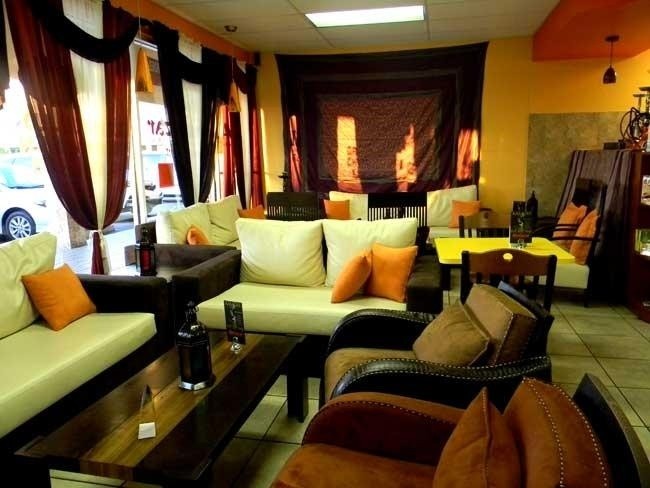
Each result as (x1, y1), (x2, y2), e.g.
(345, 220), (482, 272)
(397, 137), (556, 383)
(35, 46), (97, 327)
(431, 178), (607, 307)
(100, 258), (198, 289)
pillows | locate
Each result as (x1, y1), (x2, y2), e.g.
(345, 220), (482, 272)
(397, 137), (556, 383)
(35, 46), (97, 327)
(449, 201), (480, 228)
(234, 218), (420, 305)
(553, 200), (600, 263)
(155, 194), (267, 245)
(464, 282), (538, 366)
(324, 199), (351, 220)
(432, 376), (610, 488)
(413, 296), (491, 366)
(23, 261), (97, 331)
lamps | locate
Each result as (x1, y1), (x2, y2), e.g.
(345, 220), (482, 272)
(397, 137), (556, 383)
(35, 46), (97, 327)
(223, 24), (241, 113)
(603, 34), (619, 84)
(134, 0), (154, 93)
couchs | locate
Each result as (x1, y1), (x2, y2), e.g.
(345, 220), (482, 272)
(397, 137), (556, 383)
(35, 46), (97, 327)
(0, 231), (171, 478)
(171, 215), (443, 380)
(122, 207), (236, 266)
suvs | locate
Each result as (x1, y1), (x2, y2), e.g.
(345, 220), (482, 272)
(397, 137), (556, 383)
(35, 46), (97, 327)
(120, 171), (164, 215)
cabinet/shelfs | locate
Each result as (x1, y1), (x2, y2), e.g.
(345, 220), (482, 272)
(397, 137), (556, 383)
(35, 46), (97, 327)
(626, 150), (650, 323)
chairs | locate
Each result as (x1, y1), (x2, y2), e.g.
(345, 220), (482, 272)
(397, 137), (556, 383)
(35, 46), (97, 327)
(460, 251), (557, 314)
(426, 185), (477, 241)
(329, 190), (368, 222)
(316, 280), (555, 409)
(269, 371), (650, 488)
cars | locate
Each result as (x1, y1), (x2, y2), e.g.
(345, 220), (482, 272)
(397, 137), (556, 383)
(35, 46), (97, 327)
(1, 161), (47, 240)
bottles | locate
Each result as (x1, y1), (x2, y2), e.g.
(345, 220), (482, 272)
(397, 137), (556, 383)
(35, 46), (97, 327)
(177, 303), (216, 392)
(134, 227), (157, 276)
(527, 190), (539, 228)
